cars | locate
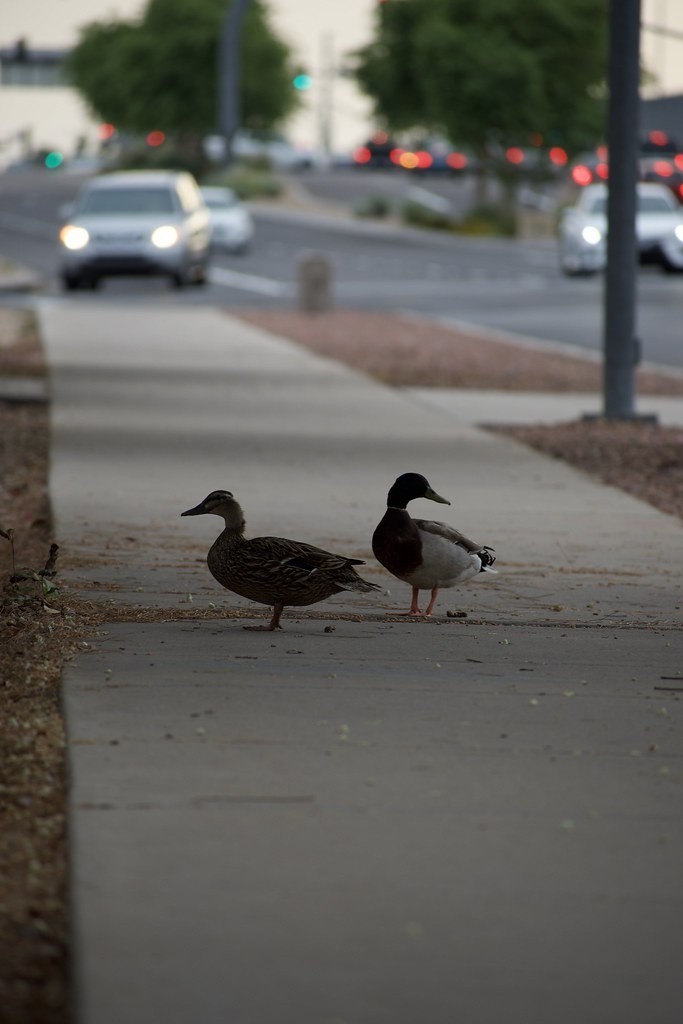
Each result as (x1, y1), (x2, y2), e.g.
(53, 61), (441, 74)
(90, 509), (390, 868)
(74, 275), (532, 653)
(199, 183), (256, 255)
(556, 179), (683, 276)
(358, 137), (466, 176)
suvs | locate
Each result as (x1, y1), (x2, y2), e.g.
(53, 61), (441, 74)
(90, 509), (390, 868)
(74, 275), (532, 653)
(51, 165), (216, 292)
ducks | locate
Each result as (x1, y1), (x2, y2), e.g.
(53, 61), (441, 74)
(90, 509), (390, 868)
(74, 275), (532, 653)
(371, 473), (496, 618)
(179, 489), (383, 632)
